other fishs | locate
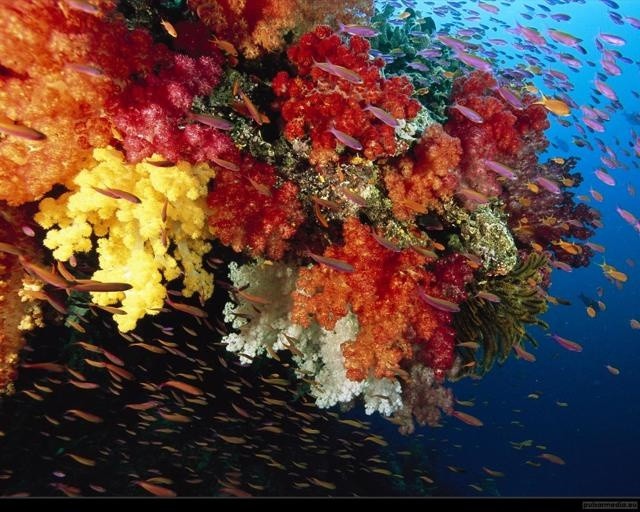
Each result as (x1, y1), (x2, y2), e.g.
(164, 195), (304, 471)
(1, 0), (640, 500)
